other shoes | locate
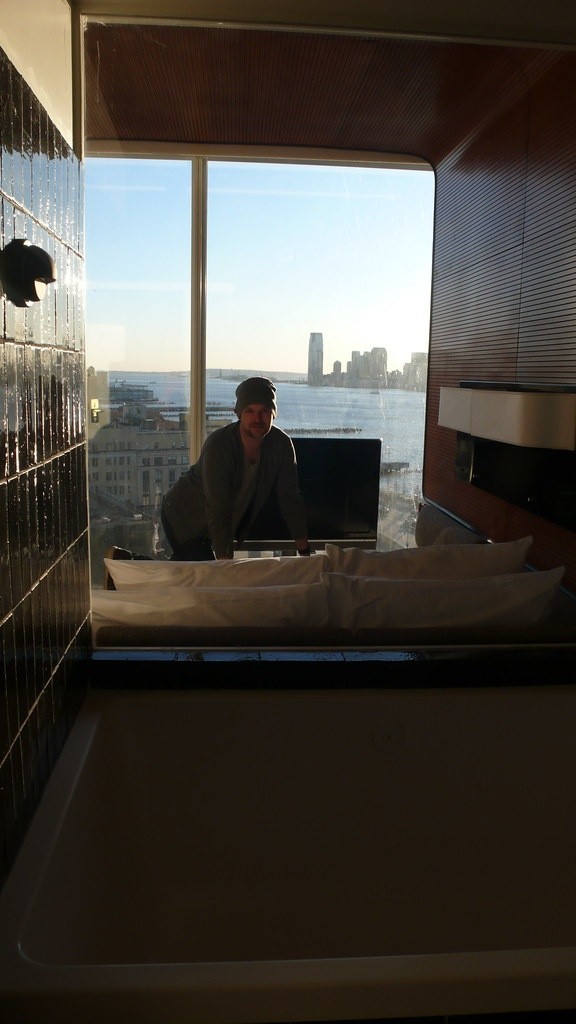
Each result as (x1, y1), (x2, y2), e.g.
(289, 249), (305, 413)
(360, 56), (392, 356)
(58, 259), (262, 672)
(105, 546), (131, 589)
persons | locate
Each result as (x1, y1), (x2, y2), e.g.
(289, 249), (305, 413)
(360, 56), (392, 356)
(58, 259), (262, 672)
(160, 376), (311, 561)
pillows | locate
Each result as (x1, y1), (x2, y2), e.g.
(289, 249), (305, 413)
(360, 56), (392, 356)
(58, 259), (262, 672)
(318, 562), (567, 628)
(324, 535), (535, 581)
(103, 552), (326, 590)
(433, 528), (485, 548)
(90, 581), (331, 647)
(414, 505), (466, 547)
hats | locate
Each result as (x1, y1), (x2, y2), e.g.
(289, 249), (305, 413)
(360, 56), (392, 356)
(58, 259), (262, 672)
(235, 378), (275, 410)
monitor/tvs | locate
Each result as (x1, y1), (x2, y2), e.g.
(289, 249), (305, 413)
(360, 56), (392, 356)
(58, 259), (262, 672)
(233, 438), (382, 552)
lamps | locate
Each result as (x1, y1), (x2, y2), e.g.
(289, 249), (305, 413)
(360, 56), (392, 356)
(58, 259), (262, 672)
(436, 380), (575, 452)
